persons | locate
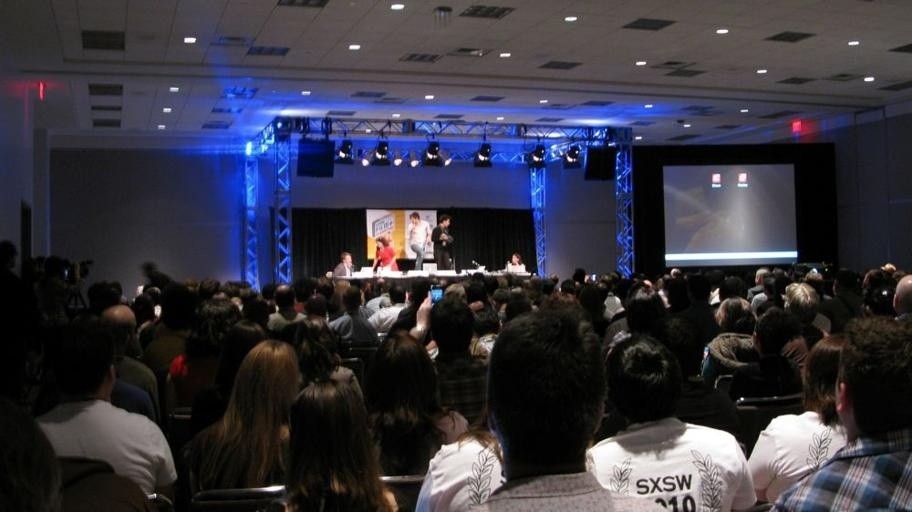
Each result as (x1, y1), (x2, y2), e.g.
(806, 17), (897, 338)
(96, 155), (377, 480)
(431, 215), (455, 270)
(0, 236), (912, 510)
(406, 212), (432, 270)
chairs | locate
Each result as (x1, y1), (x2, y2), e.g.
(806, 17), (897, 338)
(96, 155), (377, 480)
(56, 340), (806, 512)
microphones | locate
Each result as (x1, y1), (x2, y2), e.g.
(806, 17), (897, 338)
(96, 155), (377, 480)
(472, 260), (480, 268)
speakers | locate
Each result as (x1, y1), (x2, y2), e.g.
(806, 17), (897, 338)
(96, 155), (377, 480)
(296, 138), (335, 177)
(793, 261), (833, 295)
(583, 146), (616, 180)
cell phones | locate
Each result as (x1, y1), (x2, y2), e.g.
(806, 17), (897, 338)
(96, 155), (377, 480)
(431, 285), (444, 304)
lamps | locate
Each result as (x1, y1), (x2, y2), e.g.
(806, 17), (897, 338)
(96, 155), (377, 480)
(336, 134), (582, 169)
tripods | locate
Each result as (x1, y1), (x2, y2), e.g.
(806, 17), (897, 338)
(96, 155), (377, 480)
(67, 291), (87, 314)
